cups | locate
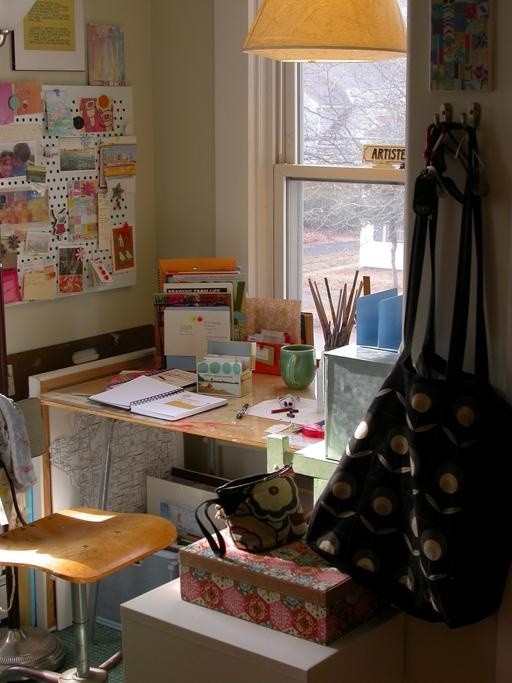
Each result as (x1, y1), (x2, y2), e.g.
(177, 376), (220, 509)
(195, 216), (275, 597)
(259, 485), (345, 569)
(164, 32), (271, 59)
(279, 345), (316, 390)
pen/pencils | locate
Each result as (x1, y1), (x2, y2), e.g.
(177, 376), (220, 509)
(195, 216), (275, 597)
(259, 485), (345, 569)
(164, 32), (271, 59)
(309, 270), (364, 349)
(272, 408), (293, 413)
(236, 403), (248, 418)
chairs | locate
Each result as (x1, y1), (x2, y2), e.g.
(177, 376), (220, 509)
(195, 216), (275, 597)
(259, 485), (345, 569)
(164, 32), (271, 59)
(1, 396), (178, 682)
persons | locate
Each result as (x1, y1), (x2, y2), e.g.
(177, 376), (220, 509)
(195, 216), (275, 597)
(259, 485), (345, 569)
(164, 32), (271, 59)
(0, 141), (36, 177)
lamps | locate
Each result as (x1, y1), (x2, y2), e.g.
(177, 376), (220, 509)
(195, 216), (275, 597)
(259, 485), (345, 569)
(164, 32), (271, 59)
(241, 0), (406, 63)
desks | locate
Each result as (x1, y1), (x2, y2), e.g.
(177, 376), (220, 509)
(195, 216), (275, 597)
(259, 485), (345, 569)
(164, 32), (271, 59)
(39, 368), (324, 631)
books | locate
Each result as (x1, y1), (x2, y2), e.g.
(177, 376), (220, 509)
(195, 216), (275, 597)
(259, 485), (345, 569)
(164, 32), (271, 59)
(87, 365), (228, 422)
(151, 258), (314, 373)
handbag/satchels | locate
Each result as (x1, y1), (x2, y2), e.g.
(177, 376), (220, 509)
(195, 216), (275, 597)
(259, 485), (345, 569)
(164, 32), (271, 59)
(194, 462), (306, 558)
(306, 354), (510, 630)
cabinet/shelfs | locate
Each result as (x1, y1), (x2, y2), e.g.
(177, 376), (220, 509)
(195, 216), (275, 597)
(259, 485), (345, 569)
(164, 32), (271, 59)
(118, 578), (406, 682)
